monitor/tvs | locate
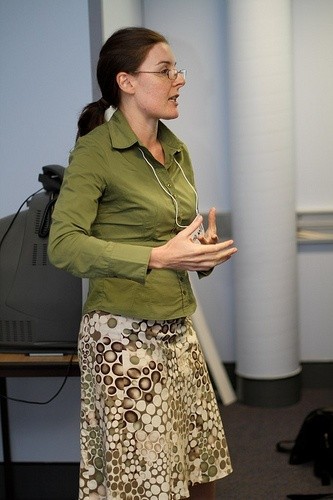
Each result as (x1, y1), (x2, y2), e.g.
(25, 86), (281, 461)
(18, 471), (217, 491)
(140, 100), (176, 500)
(0, 191), (84, 353)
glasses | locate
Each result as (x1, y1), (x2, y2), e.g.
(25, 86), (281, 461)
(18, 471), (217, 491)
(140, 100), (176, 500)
(129, 69), (187, 80)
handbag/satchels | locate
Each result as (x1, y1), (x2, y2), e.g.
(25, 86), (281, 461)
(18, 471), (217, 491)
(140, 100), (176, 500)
(277, 406), (333, 486)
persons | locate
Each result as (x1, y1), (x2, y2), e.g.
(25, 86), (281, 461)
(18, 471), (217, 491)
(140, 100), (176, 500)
(39, 24), (240, 500)
